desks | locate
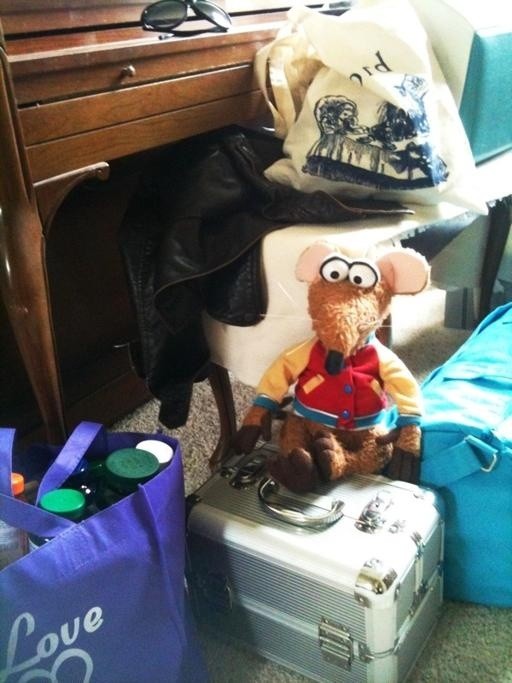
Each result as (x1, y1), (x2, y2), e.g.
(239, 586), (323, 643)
(2, 1), (349, 448)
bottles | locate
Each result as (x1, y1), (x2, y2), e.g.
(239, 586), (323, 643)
(23, 488), (87, 552)
(70, 460), (99, 519)
(100, 447), (159, 512)
(0, 471), (25, 571)
(135, 440), (172, 467)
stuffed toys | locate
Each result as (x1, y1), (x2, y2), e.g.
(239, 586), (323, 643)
(233, 239), (431, 494)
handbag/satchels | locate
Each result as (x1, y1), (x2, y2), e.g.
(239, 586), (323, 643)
(388, 301), (512, 607)
(1, 420), (207, 681)
(253, 3), (490, 215)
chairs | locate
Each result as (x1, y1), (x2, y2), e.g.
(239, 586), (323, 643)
(200, 0), (512, 467)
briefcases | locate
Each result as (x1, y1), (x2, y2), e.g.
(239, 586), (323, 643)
(184, 438), (444, 682)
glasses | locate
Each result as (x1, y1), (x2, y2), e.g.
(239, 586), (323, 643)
(140, 0), (233, 39)
